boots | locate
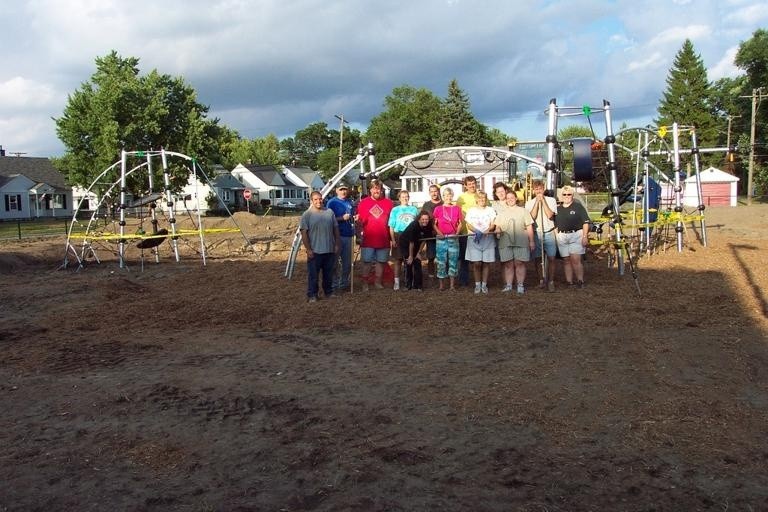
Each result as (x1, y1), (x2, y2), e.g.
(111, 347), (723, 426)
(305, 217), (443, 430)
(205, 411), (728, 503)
(393, 277), (400, 291)
(362, 276), (384, 292)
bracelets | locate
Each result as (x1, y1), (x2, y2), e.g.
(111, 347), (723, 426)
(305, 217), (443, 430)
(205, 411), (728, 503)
(582, 235), (587, 239)
(529, 240), (536, 243)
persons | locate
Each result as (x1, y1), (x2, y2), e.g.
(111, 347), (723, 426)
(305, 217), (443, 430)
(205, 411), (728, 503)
(397, 210), (434, 293)
(491, 181), (514, 216)
(355, 178), (396, 297)
(421, 184), (445, 277)
(553, 184), (591, 290)
(493, 190), (537, 295)
(524, 180), (558, 292)
(325, 181), (359, 290)
(387, 190), (421, 291)
(455, 175), (492, 287)
(463, 191), (497, 295)
(431, 187), (463, 293)
(299, 190), (341, 306)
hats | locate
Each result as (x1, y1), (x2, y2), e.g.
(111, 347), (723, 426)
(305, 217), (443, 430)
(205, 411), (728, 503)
(336, 182), (349, 190)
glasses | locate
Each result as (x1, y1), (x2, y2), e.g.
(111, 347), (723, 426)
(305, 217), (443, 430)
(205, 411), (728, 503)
(563, 194), (572, 196)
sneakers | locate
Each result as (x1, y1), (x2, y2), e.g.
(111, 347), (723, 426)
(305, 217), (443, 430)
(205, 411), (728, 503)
(518, 286), (524, 294)
(309, 279), (347, 304)
(438, 286), (457, 293)
(501, 285), (513, 292)
(402, 287), (422, 292)
(536, 279), (585, 292)
(474, 286), (488, 296)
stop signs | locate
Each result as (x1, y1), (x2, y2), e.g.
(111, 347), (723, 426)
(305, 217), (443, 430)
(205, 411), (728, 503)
(244, 189), (251, 200)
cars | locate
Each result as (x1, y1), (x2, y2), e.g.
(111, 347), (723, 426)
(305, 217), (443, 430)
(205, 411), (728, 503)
(277, 201), (296, 208)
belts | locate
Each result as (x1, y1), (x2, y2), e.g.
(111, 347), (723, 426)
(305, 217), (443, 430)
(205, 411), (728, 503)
(561, 229), (578, 233)
